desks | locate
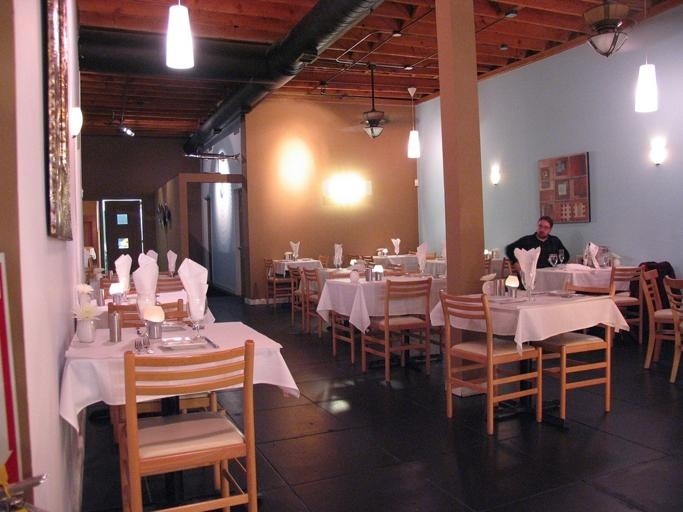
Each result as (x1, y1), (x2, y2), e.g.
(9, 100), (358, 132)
(430, 288), (629, 407)
(314, 278), (448, 365)
(60, 321), (299, 504)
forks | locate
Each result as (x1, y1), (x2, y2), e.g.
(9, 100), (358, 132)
(132, 322), (153, 356)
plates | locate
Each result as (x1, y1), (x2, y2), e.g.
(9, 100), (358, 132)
(155, 336), (207, 348)
(545, 288), (575, 296)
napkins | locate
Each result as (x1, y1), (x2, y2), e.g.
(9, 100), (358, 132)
(178, 258), (209, 319)
(147, 250), (158, 264)
(115, 254), (133, 293)
(133, 259), (160, 316)
(167, 250), (178, 273)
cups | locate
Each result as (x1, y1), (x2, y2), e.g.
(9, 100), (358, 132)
(96, 287), (128, 306)
(281, 248), (428, 283)
(493, 273), (519, 299)
(168, 270), (175, 279)
(107, 270), (113, 281)
(144, 320), (163, 339)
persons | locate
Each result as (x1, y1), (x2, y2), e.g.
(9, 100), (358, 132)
(504, 217), (569, 289)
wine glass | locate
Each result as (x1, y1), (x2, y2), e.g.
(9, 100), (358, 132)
(558, 248), (564, 266)
(521, 273), (536, 303)
(188, 302), (209, 341)
(548, 254), (558, 269)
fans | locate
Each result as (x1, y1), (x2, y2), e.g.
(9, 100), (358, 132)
(359, 65), (390, 138)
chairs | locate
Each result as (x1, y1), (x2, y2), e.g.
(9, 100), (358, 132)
(585, 265), (647, 349)
(528, 280), (616, 419)
(360, 276), (434, 381)
(328, 270), (355, 363)
(663, 274), (683, 383)
(72, 270), (214, 327)
(638, 269), (681, 368)
(438, 288), (543, 432)
(270, 236), (682, 340)
(117, 339), (260, 512)
(107, 299), (219, 442)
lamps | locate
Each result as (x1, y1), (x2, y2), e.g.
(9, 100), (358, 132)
(363, 127), (384, 139)
(583, 3), (630, 58)
(633, 0), (658, 112)
(164, 1), (195, 70)
(406, 88), (421, 159)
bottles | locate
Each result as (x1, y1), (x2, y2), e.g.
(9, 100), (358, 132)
(108, 310), (121, 341)
